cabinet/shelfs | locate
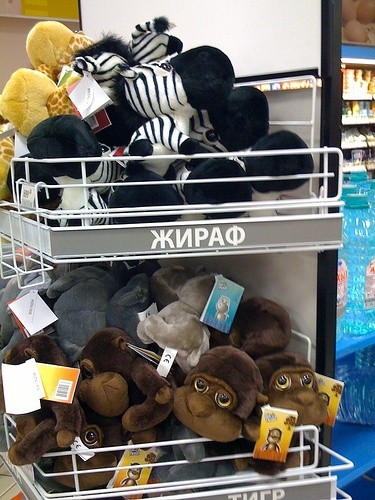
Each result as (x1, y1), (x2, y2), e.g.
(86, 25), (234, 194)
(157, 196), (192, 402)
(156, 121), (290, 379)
(1, 0), (375, 499)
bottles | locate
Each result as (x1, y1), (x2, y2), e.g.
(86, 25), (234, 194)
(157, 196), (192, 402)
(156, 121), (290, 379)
(335, 165), (375, 427)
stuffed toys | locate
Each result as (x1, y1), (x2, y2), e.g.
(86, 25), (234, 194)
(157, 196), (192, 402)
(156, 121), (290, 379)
(0, 12), (313, 227)
(0, 260), (327, 500)
